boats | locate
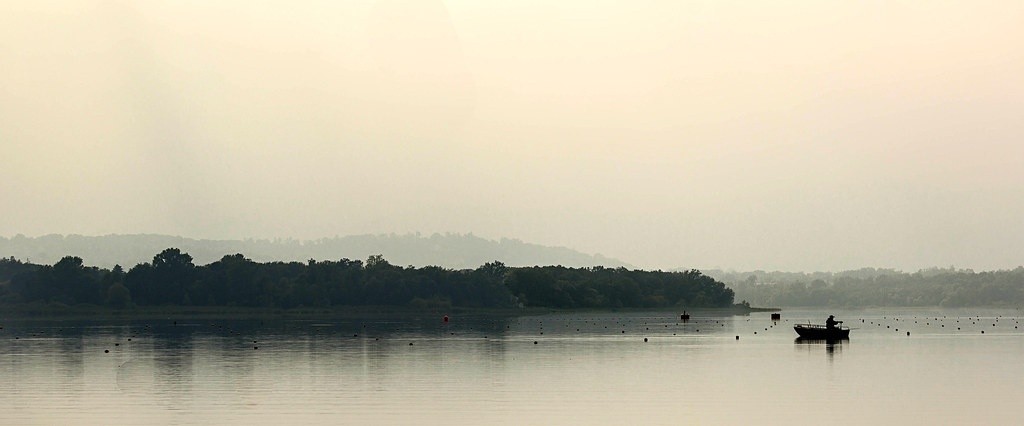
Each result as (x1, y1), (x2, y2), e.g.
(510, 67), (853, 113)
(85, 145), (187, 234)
(793, 323), (850, 339)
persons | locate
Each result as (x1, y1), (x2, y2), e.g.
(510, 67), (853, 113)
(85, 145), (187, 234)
(826, 315), (841, 329)
